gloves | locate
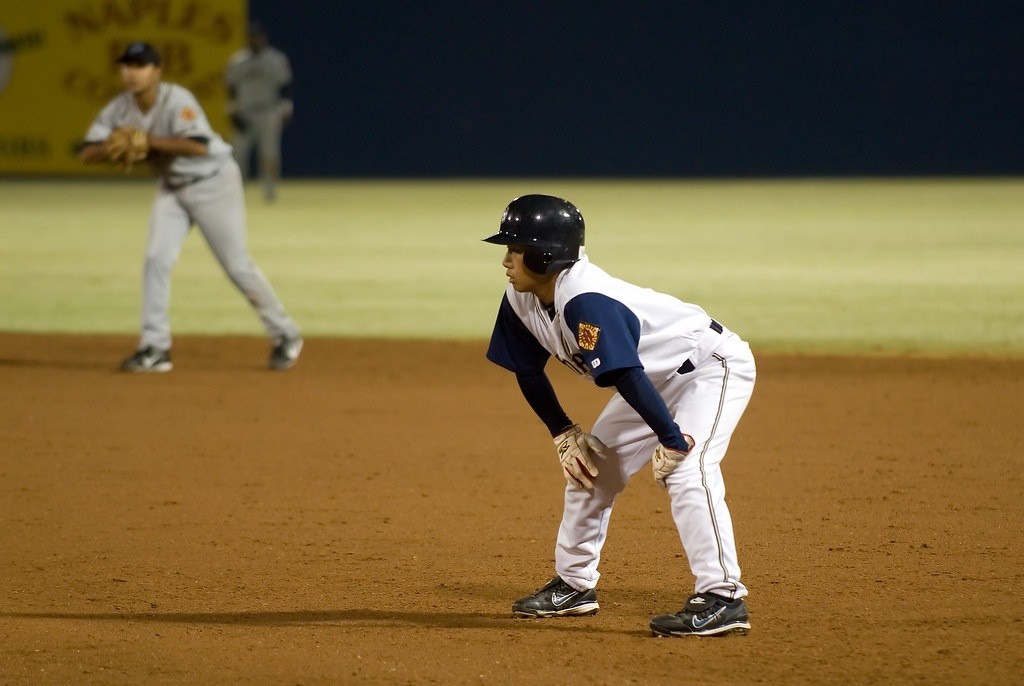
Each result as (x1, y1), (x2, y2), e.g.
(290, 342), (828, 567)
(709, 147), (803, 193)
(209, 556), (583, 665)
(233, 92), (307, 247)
(551, 423), (610, 491)
(652, 433), (695, 490)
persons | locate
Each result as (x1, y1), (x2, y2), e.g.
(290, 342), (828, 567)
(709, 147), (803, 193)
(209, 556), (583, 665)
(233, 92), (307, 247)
(227, 21), (297, 205)
(482, 194), (756, 637)
(73, 43), (306, 372)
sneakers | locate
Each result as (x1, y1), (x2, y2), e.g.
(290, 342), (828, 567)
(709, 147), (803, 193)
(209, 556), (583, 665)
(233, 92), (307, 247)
(512, 575), (600, 618)
(649, 593), (752, 637)
(120, 344), (174, 373)
(269, 334), (304, 370)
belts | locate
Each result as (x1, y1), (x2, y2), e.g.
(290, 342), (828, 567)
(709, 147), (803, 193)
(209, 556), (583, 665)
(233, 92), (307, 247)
(677, 318), (723, 375)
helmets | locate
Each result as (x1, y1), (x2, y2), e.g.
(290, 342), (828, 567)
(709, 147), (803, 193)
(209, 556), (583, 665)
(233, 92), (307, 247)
(481, 194), (585, 275)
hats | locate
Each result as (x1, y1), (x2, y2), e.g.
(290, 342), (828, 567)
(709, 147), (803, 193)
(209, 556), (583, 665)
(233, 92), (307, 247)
(113, 42), (161, 67)
